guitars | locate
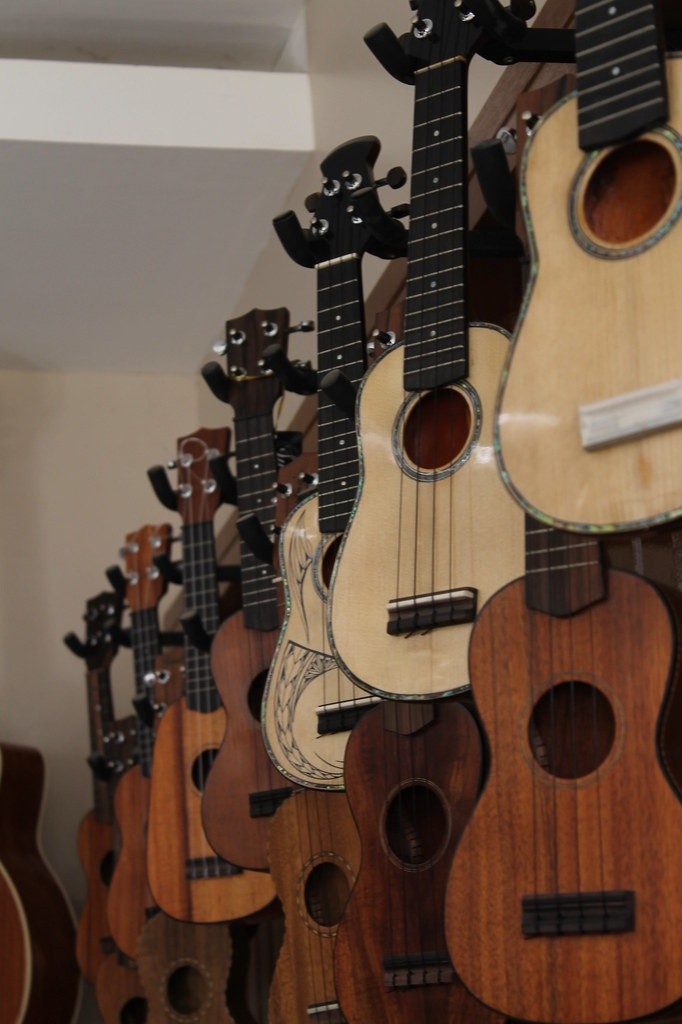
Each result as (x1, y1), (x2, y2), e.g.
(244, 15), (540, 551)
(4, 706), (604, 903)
(334, 699), (503, 1024)
(262, 135), (410, 792)
(0, 743), (80, 1024)
(105, 523), (187, 958)
(63, 565), (135, 971)
(329, 0), (539, 699)
(138, 909), (238, 1024)
(267, 788), (357, 1024)
(94, 948), (147, 1024)
(202, 308), (321, 869)
(148, 426), (277, 922)
(443, 513), (682, 1024)
(495, 0), (682, 533)
